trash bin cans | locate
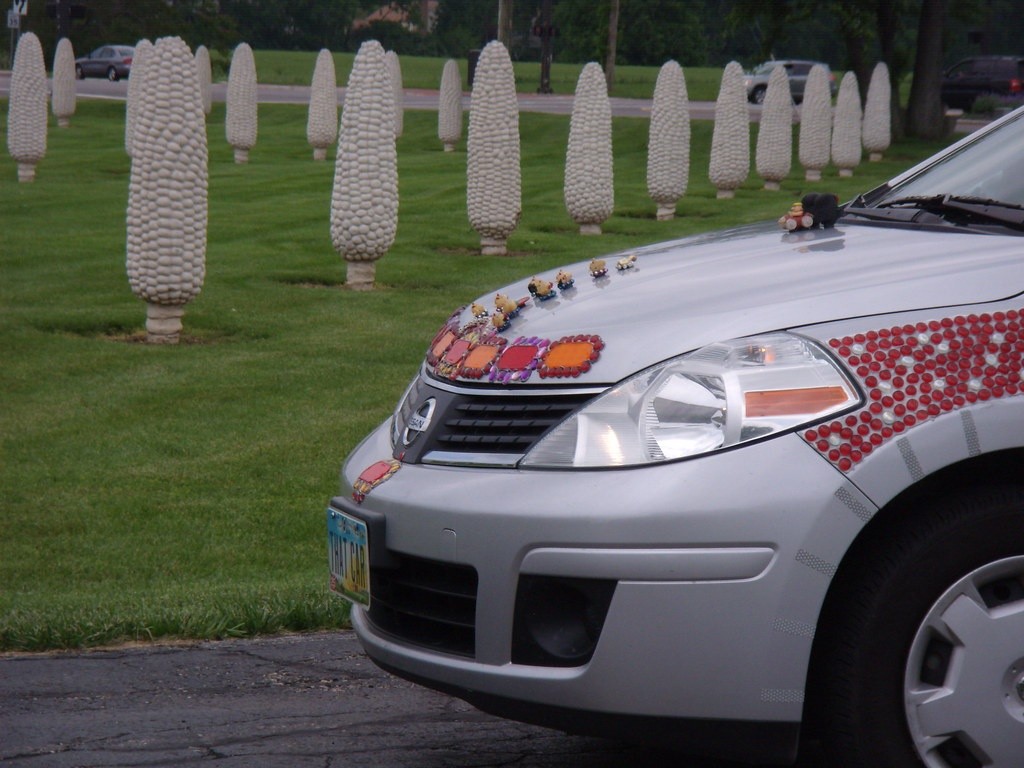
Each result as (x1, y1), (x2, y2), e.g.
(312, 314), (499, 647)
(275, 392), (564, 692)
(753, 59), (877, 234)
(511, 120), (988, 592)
(467, 47), (482, 87)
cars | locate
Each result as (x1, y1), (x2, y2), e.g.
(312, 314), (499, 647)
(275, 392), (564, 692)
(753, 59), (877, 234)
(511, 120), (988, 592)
(327, 104), (1024, 768)
(75, 45), (136, 81)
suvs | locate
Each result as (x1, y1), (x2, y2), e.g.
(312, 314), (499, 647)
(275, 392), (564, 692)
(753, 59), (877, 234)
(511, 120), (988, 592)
(743, 61), (837, 103)
(939, 57), (1023, 111)
